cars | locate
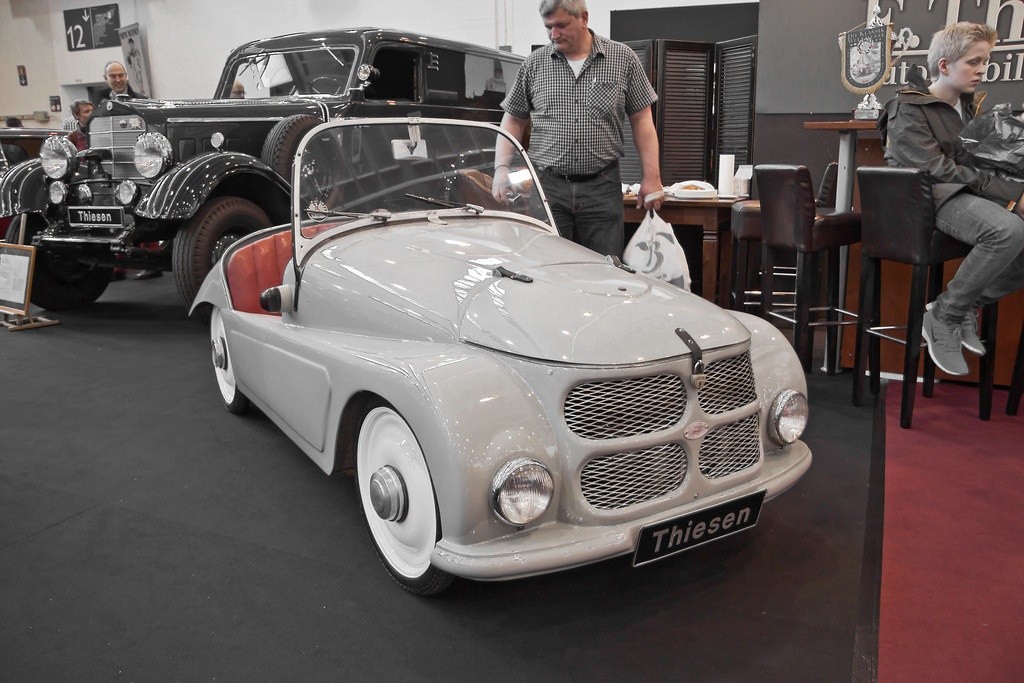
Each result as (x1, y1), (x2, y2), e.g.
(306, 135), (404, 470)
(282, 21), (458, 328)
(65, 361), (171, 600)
(186, 116), (818, 595)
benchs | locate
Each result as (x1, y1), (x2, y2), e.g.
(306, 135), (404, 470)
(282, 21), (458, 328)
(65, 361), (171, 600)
(223, 220), (357, 317)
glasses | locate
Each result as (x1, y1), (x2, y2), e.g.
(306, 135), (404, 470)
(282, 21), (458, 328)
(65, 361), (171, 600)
(232, 90), (246, 96)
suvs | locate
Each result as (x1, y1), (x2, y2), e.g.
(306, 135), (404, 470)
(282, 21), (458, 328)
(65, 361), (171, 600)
(0, 28), (535, 317)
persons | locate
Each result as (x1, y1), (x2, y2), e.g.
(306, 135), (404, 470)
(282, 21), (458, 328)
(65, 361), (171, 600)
(66, 98), (96, 151)
(874, 20), (1024, 376)
(492, 0), (665, 261)
(96, 60), (165, 280)
(229, 79), (244, 99)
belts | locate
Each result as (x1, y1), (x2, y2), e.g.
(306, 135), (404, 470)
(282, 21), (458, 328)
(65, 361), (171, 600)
(546, 159), (619, 184)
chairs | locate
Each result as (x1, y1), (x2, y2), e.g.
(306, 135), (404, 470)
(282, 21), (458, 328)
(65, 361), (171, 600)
(457, 169), (510, 212)
(731, 157), (860, 374)
(855, 165), (1000, 430)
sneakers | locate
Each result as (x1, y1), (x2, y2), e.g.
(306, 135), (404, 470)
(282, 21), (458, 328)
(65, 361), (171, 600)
(922, 310), (970, 376)
(926, 299), (987, 355)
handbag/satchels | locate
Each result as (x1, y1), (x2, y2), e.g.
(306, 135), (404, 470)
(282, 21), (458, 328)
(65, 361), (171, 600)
(623, 191), (691, 292)
(959, 102), (1024, 183)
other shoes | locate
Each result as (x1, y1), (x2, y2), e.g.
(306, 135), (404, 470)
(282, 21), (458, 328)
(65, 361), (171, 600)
(110, 272), (126, 282)
(135, 270), (164, 279)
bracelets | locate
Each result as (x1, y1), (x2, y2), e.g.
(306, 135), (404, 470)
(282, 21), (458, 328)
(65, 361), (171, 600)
(493, 164), (511, 173)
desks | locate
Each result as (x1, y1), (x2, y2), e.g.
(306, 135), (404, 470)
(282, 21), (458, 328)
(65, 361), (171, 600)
(624, 194), (746, 309)
